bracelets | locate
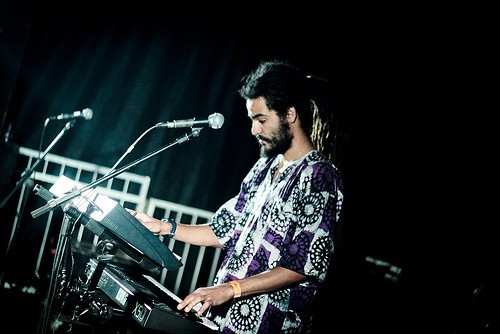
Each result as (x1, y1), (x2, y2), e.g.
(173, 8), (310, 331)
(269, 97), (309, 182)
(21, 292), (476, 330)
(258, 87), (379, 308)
(229, 280), (241, 298)
(161, 218), (177, 238)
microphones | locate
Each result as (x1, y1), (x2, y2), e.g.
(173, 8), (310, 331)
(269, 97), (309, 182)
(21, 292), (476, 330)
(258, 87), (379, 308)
(48, 108), (93, 121)
(156, 113), (224, 129)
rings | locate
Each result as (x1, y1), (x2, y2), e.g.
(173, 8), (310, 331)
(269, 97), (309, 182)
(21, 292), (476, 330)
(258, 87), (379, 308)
(132, 212), (137, 217)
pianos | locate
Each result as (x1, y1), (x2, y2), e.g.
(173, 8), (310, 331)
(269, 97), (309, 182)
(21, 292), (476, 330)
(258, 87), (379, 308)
(63, 230), (221, 334)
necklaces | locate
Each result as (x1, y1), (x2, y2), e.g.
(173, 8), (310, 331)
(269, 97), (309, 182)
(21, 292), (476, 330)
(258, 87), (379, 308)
(289, 143), (311, 163)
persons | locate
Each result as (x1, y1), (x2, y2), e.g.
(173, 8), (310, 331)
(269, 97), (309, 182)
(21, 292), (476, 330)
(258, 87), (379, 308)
(124, 61), (345, 334)
